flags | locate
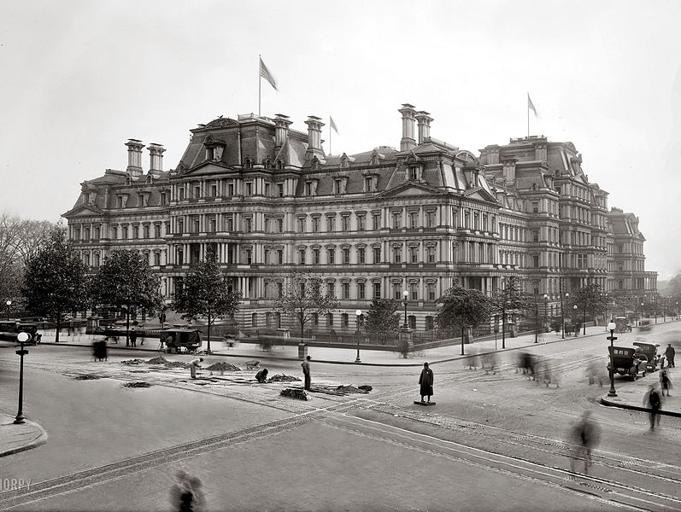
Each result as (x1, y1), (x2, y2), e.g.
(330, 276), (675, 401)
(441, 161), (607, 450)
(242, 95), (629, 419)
(330, 116), (338, 133)
(530, 96), (538, 117)
(258, 58), (279, 93)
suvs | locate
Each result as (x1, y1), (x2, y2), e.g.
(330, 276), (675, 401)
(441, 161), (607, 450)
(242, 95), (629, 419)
(607, 341), (649, 381)
(633, 341), (666, 371)
(16, 323), (41, 346)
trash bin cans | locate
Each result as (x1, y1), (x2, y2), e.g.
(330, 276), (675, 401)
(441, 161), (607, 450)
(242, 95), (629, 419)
(94, 341), (107, 361)
(298, 343), (310, 360)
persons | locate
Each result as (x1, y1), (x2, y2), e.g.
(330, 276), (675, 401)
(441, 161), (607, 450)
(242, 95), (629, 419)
(644, 382), (663, 431)
(418, 362), (435, 403)
(664, 344), (676, 367)
(255, 369), (268, 383)
(167, 466), (191, 511)
(463, 344), (607, 388)
(302, 356), (312, 389)
(190, 357), (204, 379)
(185, 474), (209, 512)
(131, 327), (138, 347)
(568, 408), (601, 476)
(659, 367), (675, 397)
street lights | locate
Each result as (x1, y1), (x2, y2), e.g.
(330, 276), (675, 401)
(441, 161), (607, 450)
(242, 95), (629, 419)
(354, 310), (364, 365)
(543, 291), (577, 338)
(12, 331), (33, 424)
(501, 299), (512, 349)
(606, 320), (621, 396)
(401, 290), (411, 328)
(641, 301), (645, 320)
(6, 300), (12, 321)
